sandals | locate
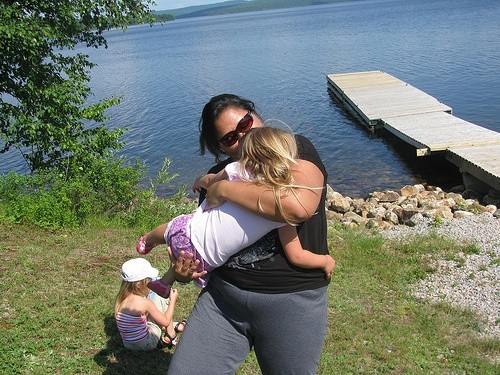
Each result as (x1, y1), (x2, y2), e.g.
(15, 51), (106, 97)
(157, 332), (176, 350)
(174, 320), (187, 332)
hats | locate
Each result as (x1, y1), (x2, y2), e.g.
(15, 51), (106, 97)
(120, 258), (159, 281)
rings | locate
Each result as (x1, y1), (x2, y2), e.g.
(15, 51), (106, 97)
(183, 267), (189, 271)
(189, 270), (195, 273)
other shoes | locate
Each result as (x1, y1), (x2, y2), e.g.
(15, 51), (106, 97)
(137, 236), (156, 255)
(147, 280), (170, 299)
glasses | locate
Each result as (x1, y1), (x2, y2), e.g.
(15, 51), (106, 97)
(217, 111), (253, 148)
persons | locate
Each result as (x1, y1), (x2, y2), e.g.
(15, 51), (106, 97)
(114, 258), (187, 353)
(137, 127), (335, 279)
(167, 94), (331, 375)
